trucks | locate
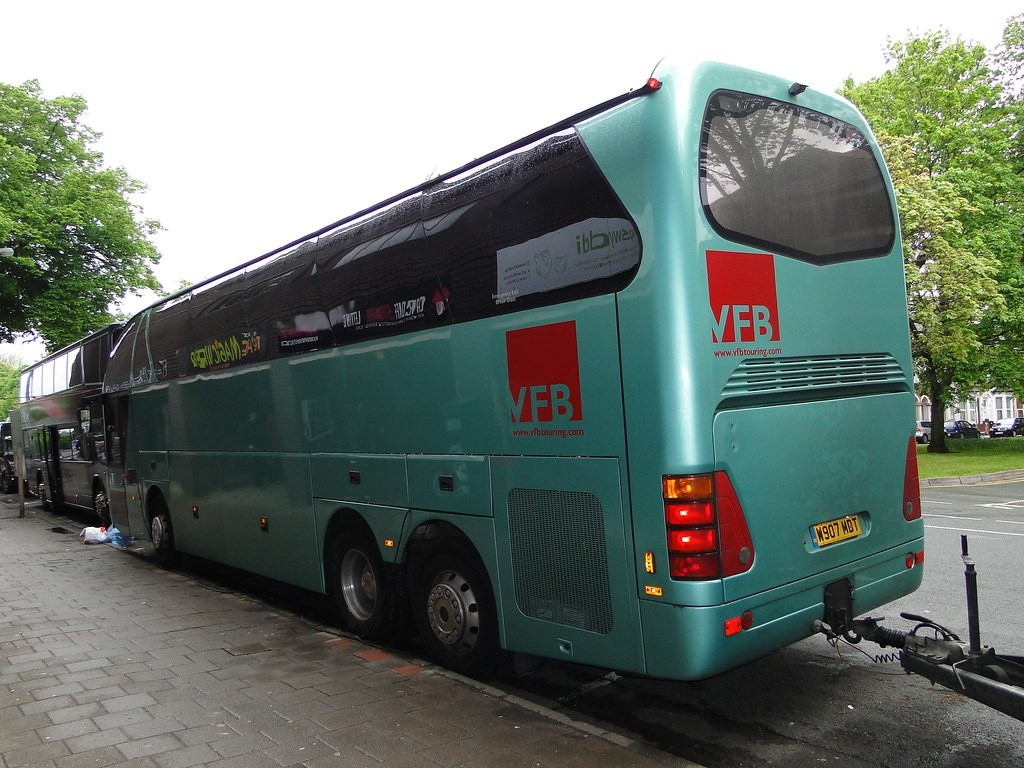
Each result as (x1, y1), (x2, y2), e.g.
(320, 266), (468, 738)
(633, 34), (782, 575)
(0, 422), (18, 494)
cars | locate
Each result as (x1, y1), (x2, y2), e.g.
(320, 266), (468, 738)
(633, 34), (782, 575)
(915, 421), (948, 444)
(988, 417), (1024, 438)
(944, 419), (981, 439)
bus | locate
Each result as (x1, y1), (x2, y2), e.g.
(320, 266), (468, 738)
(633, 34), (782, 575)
(77, 60), (923, 682)
(18, 324), (127, 532)
